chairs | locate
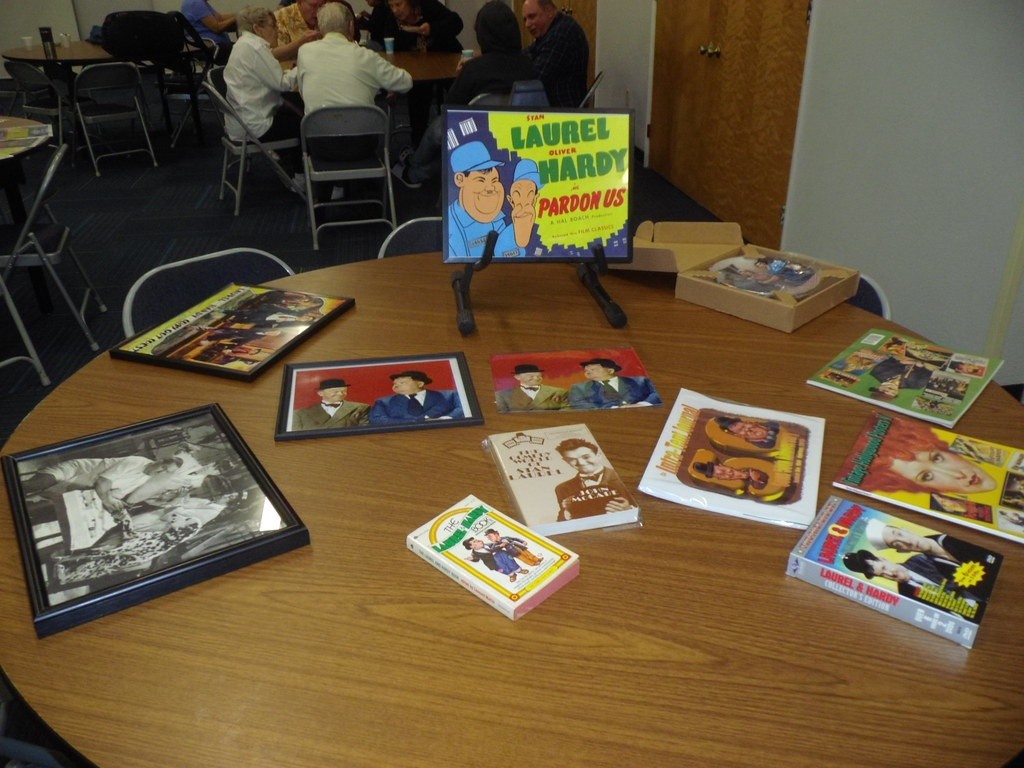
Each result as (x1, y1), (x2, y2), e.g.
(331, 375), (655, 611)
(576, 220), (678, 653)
(1, 29), (608, 390)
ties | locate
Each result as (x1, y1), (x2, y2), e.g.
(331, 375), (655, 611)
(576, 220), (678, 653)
(408, 393), (424, 416)
(603, 380), (620, 400)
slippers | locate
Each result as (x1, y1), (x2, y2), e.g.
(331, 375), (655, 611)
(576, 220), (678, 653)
(389, 161), (422, 188)
(399, 147), (419, 172)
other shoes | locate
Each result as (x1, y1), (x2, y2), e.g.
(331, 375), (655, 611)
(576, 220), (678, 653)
(291, 174), (307, 194)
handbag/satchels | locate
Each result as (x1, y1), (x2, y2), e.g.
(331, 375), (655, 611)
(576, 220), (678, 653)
(102, 11), (187, 62)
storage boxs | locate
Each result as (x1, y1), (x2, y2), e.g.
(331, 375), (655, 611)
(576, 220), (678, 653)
(608, 219), (862, 331)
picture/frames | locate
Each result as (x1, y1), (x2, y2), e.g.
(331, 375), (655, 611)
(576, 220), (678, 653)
(275, 352), (486, 440)
(110, 279), (357, 382)
(0, 403), (313, 639)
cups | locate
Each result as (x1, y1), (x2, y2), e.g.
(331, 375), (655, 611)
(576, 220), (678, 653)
(384, 37), (394, 54)
(21, 36), (32, 51)
(60, 33), (71, 48)
(462, 50), (474, 63)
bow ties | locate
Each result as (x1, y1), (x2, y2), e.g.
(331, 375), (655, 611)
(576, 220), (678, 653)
(579, 470), (606, 482)
(521, 385), (538, 391)
(322, 402), (341, 408)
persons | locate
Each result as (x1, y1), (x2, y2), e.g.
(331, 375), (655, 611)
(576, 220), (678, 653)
(568, 359), (659, 409)
(182, 0), (238, 67)
(521, 0), (589, 107)
(370, 371), (464, 426)
(358, 0), (465, 151)
(840, 411), (997, 493)
(224, 6), (343, 201)
(292, 379), (369, 431)
(269, 0), (325, 58)
(496, 364), (568, 413)
(298, 3), (412, 226)
(184, 291), (322, 356)
(394, 0), (551, 188)
(555, 438), (637, 523)
(931, 494), (968, 513)
(23, 456), (257, 592)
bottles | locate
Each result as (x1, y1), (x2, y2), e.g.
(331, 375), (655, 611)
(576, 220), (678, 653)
(39, 27), (57, 59)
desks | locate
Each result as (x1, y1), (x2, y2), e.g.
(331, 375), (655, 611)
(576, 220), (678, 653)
(0, 114), (56, 313)
(3, 41), (211, 146)
(0, 252), (1024, 768)
(378, 50), (478, 81)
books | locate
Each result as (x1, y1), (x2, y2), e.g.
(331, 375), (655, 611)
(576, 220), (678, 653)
(488, 422), (639, 536)
(407, 493), (580, 621)
(785, 494), (1003, 648)
(833, 409), (1024, 543)
(806, 329), (1005, 429)
(639, 387), (826, 530)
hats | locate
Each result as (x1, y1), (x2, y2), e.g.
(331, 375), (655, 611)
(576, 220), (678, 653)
(844, 550), (877, 580)
(390, 371), (432, 385)
(582, 358), (622, 372)
(694, 462), (714, 477)
(866, 518), (890, 551)
(512, 364), (543, 374)
(317, 379), (350, 391)
(869, 387), (893, 402)
